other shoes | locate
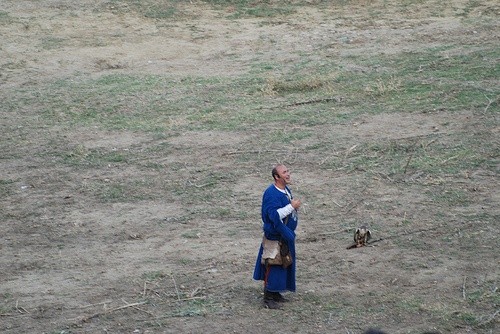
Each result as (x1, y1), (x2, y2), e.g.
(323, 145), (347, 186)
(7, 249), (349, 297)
(264, 294), (280, 309)
(273, 294), (289, 302)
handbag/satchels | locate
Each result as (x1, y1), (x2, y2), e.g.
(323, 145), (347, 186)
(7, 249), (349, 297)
(262, 238), (282, 265)
(281, 244), (293, 268)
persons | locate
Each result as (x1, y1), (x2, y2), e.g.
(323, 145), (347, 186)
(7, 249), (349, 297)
(252, 164), (301, 309)
